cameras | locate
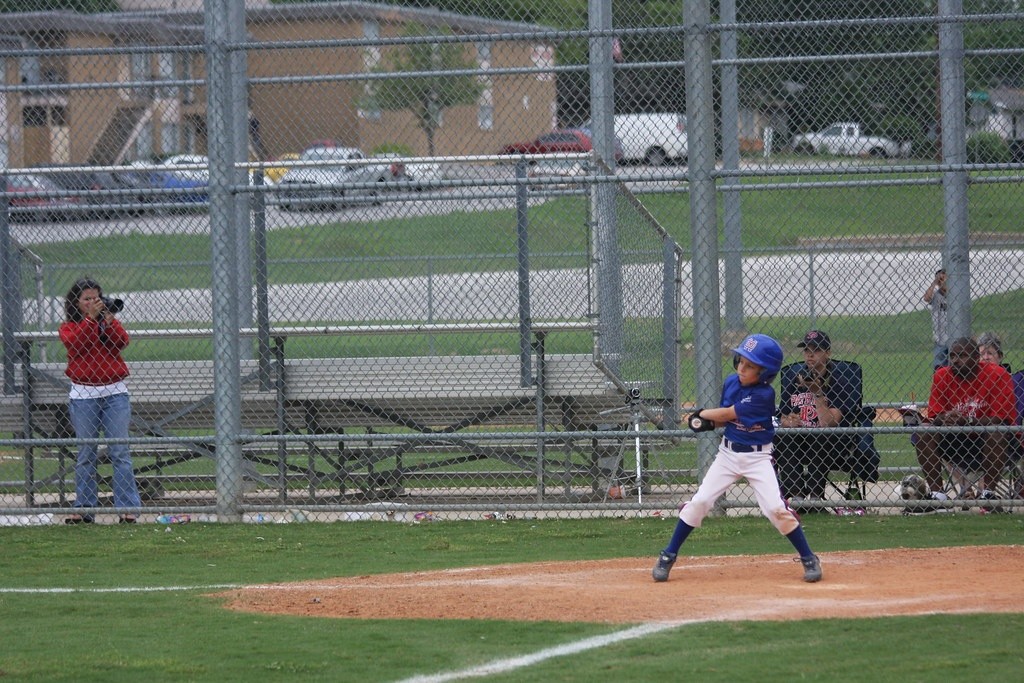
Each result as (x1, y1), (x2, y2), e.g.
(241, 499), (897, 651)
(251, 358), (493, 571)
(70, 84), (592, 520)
(799, 370), (818, 382)
(99, 298), (125, 318)
(630, 388), (641, 399)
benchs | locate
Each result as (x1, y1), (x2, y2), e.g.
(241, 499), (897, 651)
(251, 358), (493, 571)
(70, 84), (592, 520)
(0, 353), (678, 501)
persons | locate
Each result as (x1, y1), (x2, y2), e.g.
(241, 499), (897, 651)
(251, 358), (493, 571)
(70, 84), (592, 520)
(977, 330), (1011, 373)
(773, 330), (848, 514)
(923, 269), (948, 368)
(652, 334), (822, 582)
(904, 337), (1024, 516)
(58, 279), (142, 524)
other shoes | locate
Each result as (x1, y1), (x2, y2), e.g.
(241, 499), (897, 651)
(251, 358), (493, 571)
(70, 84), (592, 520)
(65, 514), (94, 523)
(904, 492), (955, 514)
(970, 490), (1005, 512)
(119, 513), (136, 523)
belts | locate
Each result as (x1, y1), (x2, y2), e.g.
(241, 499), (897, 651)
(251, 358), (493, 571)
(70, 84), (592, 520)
(725, 439), (762, 453)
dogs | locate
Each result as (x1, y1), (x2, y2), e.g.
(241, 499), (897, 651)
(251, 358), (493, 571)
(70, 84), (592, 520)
(900, 474), (1004, 515)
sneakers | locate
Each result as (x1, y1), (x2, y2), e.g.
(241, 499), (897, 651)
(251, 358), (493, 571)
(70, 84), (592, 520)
(793, 554), (821, 582)
(652, 550), (676, 581)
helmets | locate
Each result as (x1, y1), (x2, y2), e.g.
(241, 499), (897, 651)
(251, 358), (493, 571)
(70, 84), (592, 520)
(732, 334), (783, 386)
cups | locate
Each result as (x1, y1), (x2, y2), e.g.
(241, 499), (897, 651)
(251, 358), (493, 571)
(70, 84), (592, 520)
(902, 405), (917, 426)
(607, 485), (627, 499)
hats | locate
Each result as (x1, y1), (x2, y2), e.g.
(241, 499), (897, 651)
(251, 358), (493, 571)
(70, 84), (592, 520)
(797, 331), (831, 349)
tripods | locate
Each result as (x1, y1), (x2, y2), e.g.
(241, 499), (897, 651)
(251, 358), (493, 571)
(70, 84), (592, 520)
(602, 400), (677, 504)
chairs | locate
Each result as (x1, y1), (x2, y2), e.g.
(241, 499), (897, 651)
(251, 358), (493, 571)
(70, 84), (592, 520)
(780, 358), (882, 514)
(897, 369), (1024, 512)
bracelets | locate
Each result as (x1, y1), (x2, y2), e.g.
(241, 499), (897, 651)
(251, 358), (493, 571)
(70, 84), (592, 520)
(86, 317), (95, 325)
